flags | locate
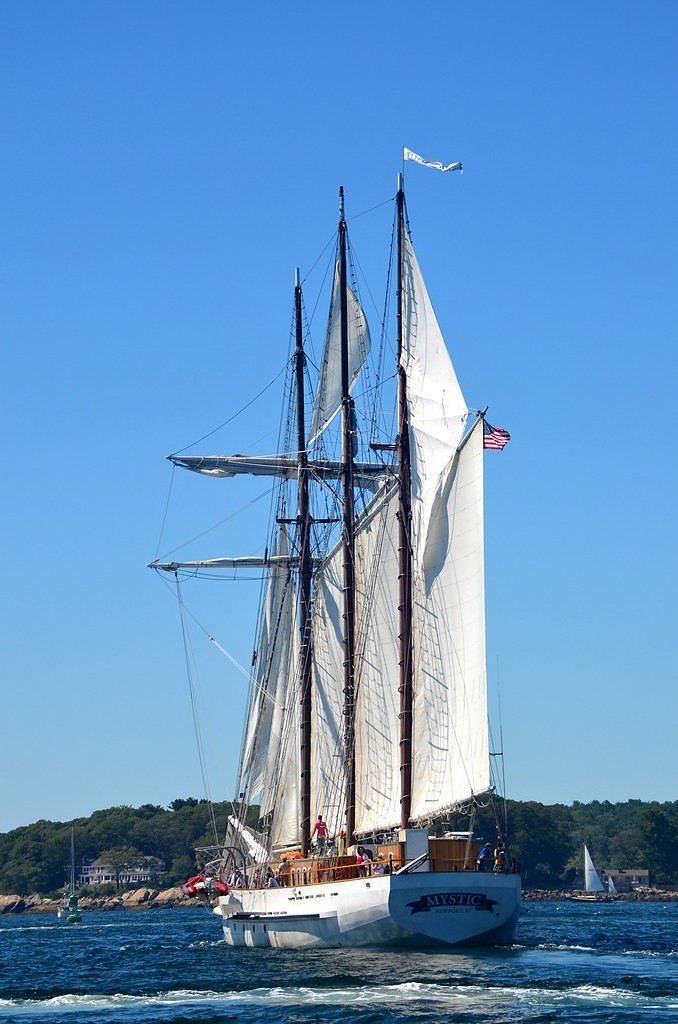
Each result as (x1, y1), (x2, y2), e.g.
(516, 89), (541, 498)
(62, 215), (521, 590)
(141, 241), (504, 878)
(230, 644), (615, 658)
(404, 148), (463, 174)
(483, 418), (512, 451)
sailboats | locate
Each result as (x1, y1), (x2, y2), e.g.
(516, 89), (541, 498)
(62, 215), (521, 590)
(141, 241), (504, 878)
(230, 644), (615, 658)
(146, 173), (522, 951)
(57, 827), (81, 917)
(566, 845), (620, 902)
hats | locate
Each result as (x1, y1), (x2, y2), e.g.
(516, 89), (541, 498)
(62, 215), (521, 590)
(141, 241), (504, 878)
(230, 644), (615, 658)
(266, 872), (271, 876)
(282, 857), (287, 860)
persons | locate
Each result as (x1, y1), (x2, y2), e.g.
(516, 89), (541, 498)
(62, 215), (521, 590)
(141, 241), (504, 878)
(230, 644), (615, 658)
(492, 843), (506, 872)
(475, 843), (492, 870)
(307, 815), (329, 855)
(356, 846), (384, 877)
(227, 867), (246, 889)
(266, 857), (290, 888)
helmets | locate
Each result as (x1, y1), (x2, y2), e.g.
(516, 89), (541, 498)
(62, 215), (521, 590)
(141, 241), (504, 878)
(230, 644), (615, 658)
(235, 869), (239, 872)
